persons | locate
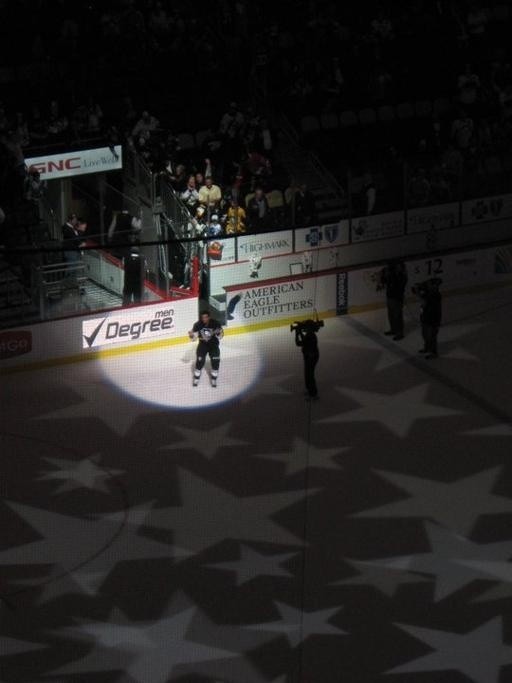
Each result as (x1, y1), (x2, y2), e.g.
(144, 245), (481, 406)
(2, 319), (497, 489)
(295, 320), (319, 398)
(384, 259), (407, 340)
(188, 313), (224, 379)
(418, 289), (442, 359)
(1, 102), (315, 306)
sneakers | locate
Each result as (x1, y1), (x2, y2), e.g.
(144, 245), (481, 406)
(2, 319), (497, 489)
(384, 330), (438, 359)
(194, 369), (218, 378)
